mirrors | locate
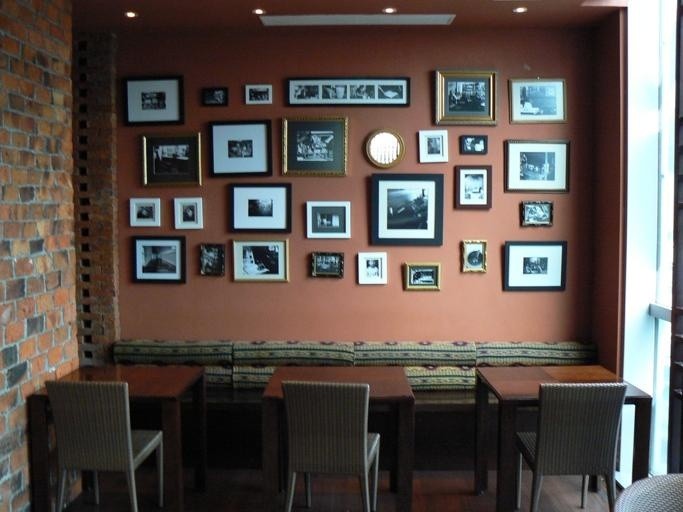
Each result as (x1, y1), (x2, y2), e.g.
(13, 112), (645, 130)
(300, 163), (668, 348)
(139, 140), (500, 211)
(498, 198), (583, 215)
(365, 129), (406, 169)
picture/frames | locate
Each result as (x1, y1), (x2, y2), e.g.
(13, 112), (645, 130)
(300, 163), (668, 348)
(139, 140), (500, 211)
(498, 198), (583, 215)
(141, 133), (203, 188)
(123, 76), (183, 125)
(281, 116), (348, 177)
(245, 84), (273, 105)
(508, 78), (568, 124)
(418, 129), (450, 164)
(209, 119), (272, 177)
(200, 243), (226, 275)
(129, 198), (161, 227)
(311, 252), (344, 278)
(461, 240), (488, 272)
(460, 135), (488, 154)
(435, 71), (498, 126)
(230, 183), (291, 232)
(307, 201), (352, 239)
(371, 173), (443, 245)
(455, 166), (492, 210)
(174, 197), (204, 229)
(357, 250), (389, 285)
(505, 240), (567, 291)
(132, 236), (186, 282)
(504, 140), (570, 194)
(288, 77), (410, 106)
(520, 202), (553, 227)
(404, 262), (440, 291)
(232, 239), (289, 283)
(201, 87), (228, 106)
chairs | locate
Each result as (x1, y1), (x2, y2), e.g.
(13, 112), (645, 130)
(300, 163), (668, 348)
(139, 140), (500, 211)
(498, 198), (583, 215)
(46, 380), (163, 511)
(518, 382), (627, 512)
(614, 472), (683, 512)
(280, 381), (380, 511)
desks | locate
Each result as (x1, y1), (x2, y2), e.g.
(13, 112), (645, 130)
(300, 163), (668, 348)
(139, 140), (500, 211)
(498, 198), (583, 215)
(474, 366), (652, 511)
(29, 363), (208, 512)
(263, 366), (415, 511)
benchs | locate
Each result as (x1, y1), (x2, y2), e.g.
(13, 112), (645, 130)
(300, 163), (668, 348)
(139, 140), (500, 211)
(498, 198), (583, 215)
(113, 340), (598, 473)
